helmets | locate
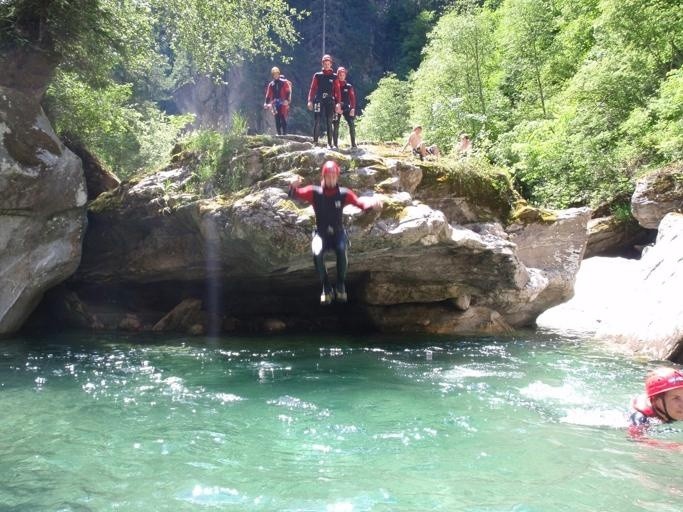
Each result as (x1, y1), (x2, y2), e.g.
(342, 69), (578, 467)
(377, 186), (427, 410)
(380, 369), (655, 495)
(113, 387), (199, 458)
(322, 161), (340, 176)
(337, 67), (347, 74)
(322, 55), (332, 62)
(646, 368), (683, 398)
(272, 67), (280, 74)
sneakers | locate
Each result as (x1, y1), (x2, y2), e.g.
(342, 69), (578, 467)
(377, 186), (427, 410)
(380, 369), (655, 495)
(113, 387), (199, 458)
(320, 284), (347, 304)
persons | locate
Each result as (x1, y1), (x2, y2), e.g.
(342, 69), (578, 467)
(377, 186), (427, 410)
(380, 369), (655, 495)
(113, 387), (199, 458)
(334, 66), (357, 149)
(453, 133), (471, 164)
(621, 364), (683, 456)
(263, 67), (292, 136)
(306, 55), (342, 150)
(287, 160), (385, 305)
(399, 126), (439, 163)
